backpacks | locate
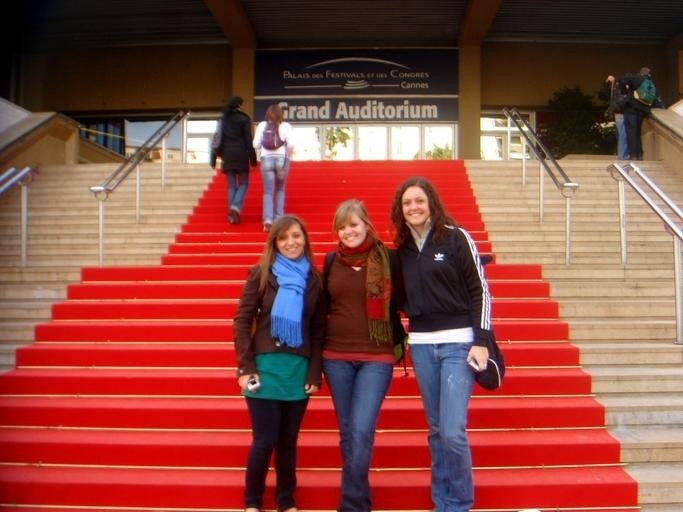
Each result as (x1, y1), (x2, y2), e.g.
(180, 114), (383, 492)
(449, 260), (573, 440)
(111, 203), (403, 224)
(261, 123), (286, 150)
(634, 79), (656, 107)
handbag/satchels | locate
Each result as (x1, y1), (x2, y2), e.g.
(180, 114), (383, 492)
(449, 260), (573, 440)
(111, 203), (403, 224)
(211, 133), (221, 152)
(475, 326), (505, 389)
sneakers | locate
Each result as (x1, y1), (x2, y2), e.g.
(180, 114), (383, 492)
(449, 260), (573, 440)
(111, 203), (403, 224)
(227, 206), (239, 224)
(263, 220), (272, 231)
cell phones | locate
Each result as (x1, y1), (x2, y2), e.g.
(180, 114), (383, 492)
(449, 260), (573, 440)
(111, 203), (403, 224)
(247, 379), (260, 392)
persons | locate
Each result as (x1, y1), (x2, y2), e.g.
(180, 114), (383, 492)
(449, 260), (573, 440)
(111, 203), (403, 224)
(390, 177), (490, 511)
(209, 96), (256, 224)
(614, 72), (633, 161)
(316, 196), (403, 512)
(252, 104), (292, 231)
(229, 211), (328, 511)
(618, 66), (664, 162)
